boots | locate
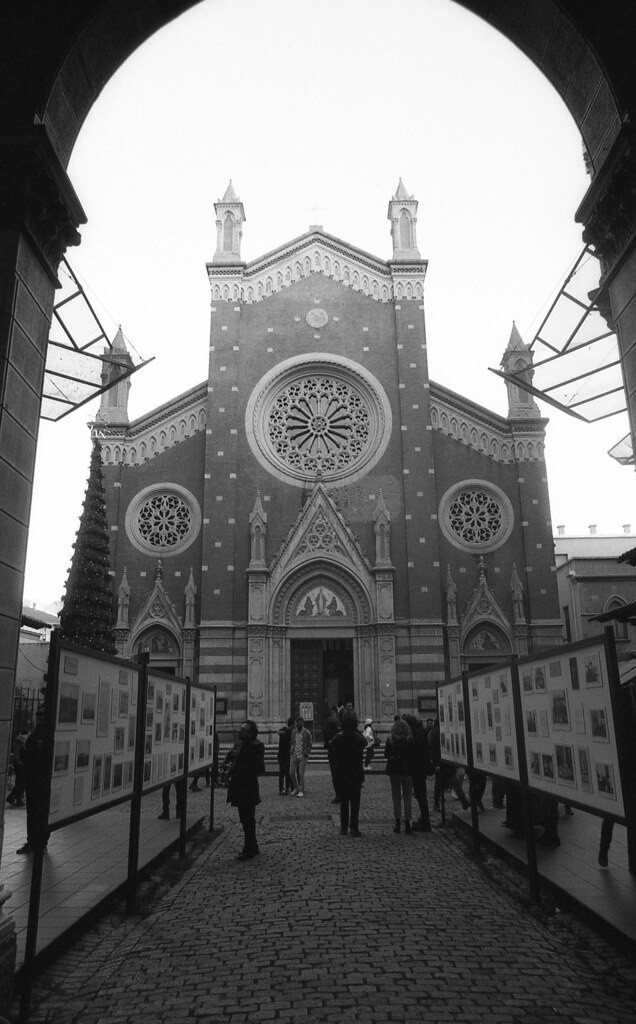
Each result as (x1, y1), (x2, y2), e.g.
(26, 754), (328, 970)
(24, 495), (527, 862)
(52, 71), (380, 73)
(405, 819), (411, 833)
(393, 820), (400, 833)
(433, 799), (440, 812)
(460, 795), (472, 811)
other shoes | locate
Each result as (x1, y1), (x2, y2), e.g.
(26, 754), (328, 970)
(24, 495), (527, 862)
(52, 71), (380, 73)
(566, 810), (573, 815)
(297, 791), (303, 797)
(411, 820), (431, 831)
(351, 829), (361, 836)
(158, 810), (169, 819)
(17, 843), (29, 854)
(340, 825), (347, 834)
(331, 797), (340, 804)
(17, 801), (24, 807)
(6, 797), (16, 806)
(291, 789), (297, 796)
(239, 845), (260, 855)
(599, 853), (608, 867)
(236, 849), (253, 859)
(365, 767), (371, 770)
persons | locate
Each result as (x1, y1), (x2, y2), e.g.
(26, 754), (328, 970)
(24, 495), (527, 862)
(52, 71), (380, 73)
(598, 818), (615, 870)
(221, 720), (266, 860)
(383, 713), (575, 845)
(277, 717), (312, 797)
(321, 696), (376, 837)
(158, 777), (181, 820)
(6, 706), (49, 855)
(188, 770), (204, 791)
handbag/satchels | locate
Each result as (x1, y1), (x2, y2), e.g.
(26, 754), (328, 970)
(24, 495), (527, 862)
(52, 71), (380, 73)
(214, 766), (231, 788)
(373, 732), (381, 747)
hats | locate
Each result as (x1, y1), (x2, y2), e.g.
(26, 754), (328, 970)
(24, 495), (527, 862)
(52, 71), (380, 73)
(366, 718), (372, 724)
